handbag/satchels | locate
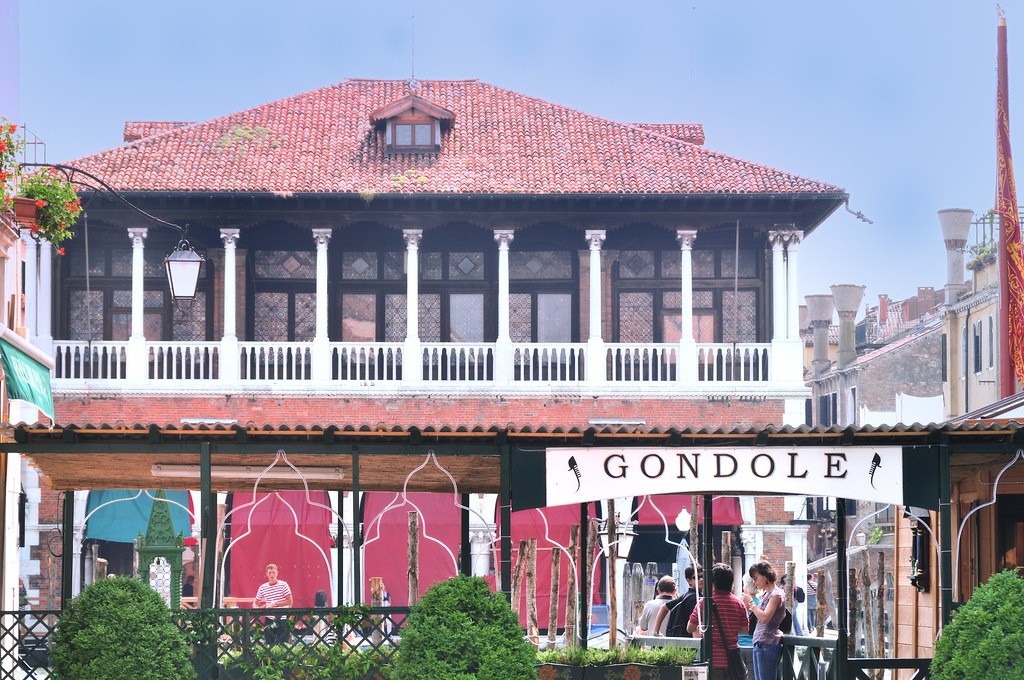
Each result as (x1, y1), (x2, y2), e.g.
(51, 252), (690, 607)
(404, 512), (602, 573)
(748, 607), (792, 635)
(726, 647), (749, 680)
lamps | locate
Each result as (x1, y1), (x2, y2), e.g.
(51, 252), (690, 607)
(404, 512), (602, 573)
(18, 163), (205, 314)
(152, 462), (344, 481)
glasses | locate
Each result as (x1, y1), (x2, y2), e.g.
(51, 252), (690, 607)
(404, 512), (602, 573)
(753, 574), (761, 580)
(690, 574), (703, 580)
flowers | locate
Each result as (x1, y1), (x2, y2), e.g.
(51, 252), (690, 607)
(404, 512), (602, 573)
(0, 121), (26, 215)
(16, 167), (84, 257)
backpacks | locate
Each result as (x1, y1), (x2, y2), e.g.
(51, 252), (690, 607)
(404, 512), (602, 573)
(794, 586), (805, 603)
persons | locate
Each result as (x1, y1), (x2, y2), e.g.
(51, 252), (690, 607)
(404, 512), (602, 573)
(253, 562), (294, 643)
(634, 560), (818, 680)
(180, 576), (195, 630)
(18, 579), (28, 634)
(378, 582), (392, 636)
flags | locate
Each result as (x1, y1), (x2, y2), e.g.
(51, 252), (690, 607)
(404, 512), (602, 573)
(0, 338), (56, 428)
(994, 80), (1024, 390)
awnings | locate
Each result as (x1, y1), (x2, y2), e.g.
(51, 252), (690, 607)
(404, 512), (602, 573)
(638, 495), (744, 525)
(82, 489), (192, 547)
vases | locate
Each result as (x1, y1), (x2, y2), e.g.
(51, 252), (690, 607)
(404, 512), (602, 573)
(14, 197), (40, 229)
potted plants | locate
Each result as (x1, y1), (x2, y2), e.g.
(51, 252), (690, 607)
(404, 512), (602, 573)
(534, 644), (697, 680)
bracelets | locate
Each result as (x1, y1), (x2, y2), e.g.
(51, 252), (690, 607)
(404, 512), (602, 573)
(748, 601), (756, 610)
(271, 601), (275, 607)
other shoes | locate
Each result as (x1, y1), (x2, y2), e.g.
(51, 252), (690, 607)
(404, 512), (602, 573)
(809, 628), (814, 633)
